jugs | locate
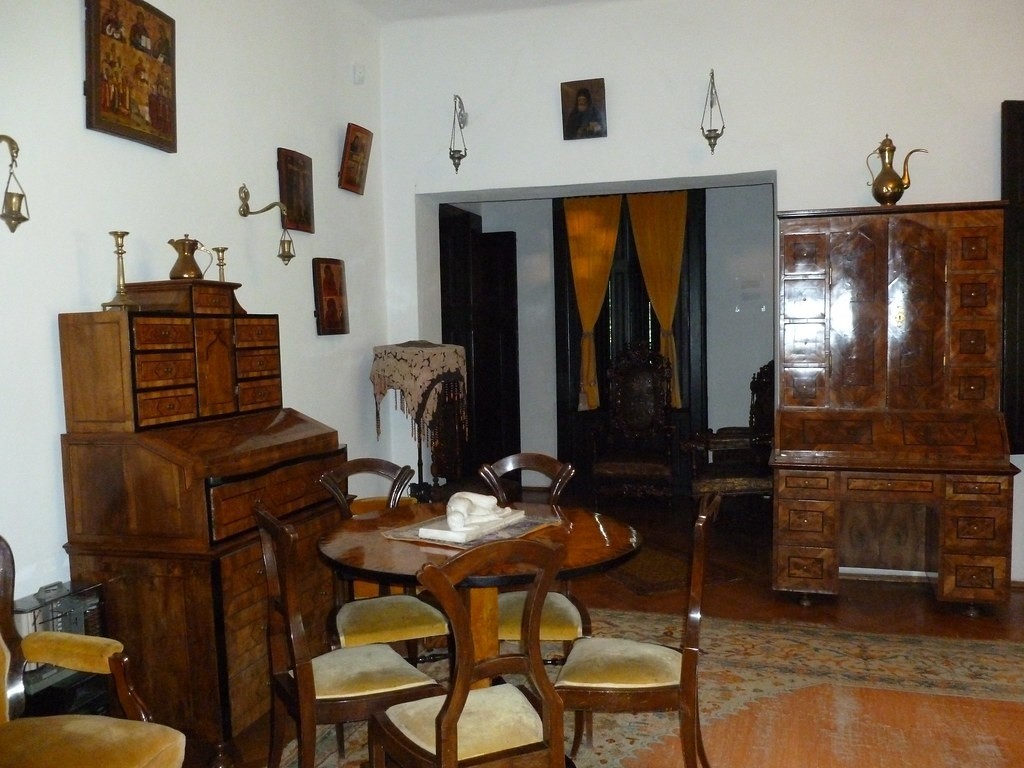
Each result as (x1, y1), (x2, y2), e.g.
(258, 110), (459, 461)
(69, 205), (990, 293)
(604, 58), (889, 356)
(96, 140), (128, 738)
(167, 234), (213, 279)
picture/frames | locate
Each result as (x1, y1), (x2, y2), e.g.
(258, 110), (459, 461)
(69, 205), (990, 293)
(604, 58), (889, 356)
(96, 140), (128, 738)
(338, 122), (373, 195)
(277, 147), (315, 234)
(83, 0), (177, 153)
(312, 258), (349, 335)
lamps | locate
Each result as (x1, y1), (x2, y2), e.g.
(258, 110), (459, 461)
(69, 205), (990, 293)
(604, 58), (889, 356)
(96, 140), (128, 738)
(369, 340), (469, 503)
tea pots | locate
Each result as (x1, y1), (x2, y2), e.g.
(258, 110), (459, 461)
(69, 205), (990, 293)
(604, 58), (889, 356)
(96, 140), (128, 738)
(866, 133), (928, 207)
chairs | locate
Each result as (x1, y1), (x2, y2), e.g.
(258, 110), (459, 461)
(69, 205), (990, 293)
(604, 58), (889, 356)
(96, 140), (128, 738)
(680, 359), (774, 529)
(588, 340), (681, 513)
(0, 534), (186, 768)
(554, 491), (724, 768)
(318, 458), (456, 684)
(252, 498), (448, 768)
(366, 538), (569, 768)
(479, 452), (594, 746)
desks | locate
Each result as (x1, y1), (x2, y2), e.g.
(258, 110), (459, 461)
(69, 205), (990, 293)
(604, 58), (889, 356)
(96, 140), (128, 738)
(316, 500), (646, 760)
(430, 475), (521, 503)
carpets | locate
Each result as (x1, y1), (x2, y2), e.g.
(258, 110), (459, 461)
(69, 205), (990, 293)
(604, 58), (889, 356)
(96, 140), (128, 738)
(276, 605), (1024, 768)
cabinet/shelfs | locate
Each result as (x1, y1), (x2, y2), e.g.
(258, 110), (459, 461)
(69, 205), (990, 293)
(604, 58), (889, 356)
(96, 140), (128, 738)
(768, 200), (1022, 616)
(57, 278), (357, 768)
